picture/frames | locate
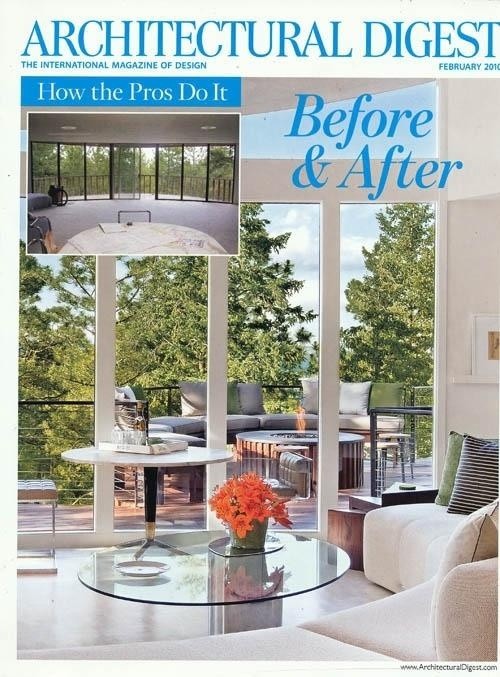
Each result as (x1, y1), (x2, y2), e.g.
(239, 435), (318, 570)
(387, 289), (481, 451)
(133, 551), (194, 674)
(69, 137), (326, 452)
(470, 311), (500, 375)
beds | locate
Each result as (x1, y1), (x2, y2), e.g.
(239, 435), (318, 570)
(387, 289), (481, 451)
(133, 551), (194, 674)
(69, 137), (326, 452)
(28, 192), (52, 211)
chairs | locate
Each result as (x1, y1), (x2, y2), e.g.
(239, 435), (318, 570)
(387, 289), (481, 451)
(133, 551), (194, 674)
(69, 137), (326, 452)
(27, 212), (55, 253)
(262, 456), (313, 504)
(117, 209), (152, 223)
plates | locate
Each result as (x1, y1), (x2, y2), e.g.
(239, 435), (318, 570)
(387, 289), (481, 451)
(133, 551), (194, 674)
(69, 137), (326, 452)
(117, 573), (170, 586)
(114, 560), (171, 576)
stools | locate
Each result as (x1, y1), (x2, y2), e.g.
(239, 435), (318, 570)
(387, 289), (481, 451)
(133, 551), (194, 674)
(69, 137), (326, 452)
(365, 443), (401, 494)
(18, 481), (58, 575)
(378, 433), (415, 483)
(272, 446), (309, 481)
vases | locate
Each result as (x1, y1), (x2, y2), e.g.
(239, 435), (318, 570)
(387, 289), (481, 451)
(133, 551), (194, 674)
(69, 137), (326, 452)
(225, 517), (271, 553)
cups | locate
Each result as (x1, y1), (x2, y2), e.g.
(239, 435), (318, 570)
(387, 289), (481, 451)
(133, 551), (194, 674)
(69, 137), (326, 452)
(111, 429), (146, 445)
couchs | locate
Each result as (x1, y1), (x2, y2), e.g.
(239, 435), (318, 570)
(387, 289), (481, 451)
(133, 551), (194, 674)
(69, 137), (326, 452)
(18, 440), (498, 661)
(111, 380), (411, 503)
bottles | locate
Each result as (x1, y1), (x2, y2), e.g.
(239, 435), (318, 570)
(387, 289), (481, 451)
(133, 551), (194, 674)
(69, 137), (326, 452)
(132, 400), (146, 431)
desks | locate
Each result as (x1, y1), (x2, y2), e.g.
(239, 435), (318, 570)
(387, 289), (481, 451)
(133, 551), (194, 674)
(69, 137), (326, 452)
(54, 223), (229, 253)
(233, 430), (366, 491)
(58, 445), (236, 567)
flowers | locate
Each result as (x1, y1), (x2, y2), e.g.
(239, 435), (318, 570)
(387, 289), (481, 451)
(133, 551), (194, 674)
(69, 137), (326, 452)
(206, 473), (296, 538)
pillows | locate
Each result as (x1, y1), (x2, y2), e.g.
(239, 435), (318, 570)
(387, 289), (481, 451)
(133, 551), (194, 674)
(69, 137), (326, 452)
(435, 428), (462, 507)
(180, 381), (207, 415)
(114, 383), (150, 424)
(430, 499), (497, 631)
(339, 380), (373, 416)
(238, 382), (269, 415)
(226, 380), (243, 414)
(370, 381), (406, 417)
(300, 375), (318, 415)
(447, 433), (498, 517)
